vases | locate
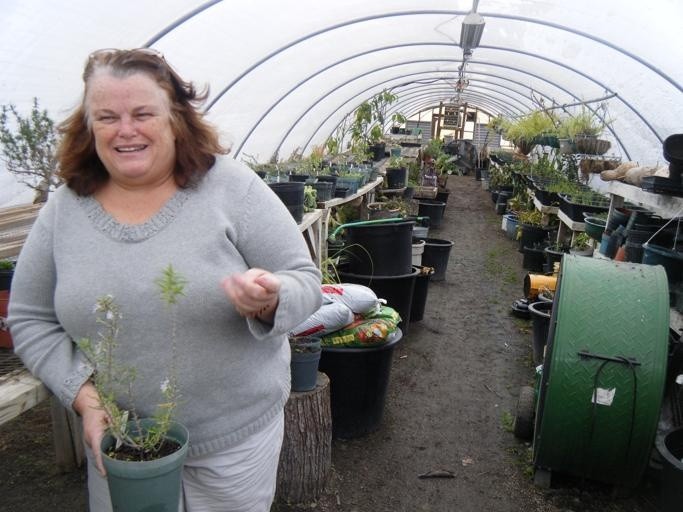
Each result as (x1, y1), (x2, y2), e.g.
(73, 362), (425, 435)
(288, 334), (321, 392)
(654, 425), (683, 512)
(585, 203), (681, 291)
(317, 199), (454, 441)
(526, 301), (554, 365)
(267, 181), (304, 225)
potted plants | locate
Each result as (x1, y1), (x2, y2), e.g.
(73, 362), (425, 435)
(490, 103), (614, 273)
(432, 153), (459, 216)
(72, 262), (193, 512)
(314, 126), (408, 194)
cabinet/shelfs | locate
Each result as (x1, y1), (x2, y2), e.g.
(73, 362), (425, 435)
(594, 174), (683, 346)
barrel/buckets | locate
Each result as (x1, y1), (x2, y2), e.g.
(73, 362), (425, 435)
(413, 225), (429, 238)
(422, 238), (454, 282)
(643, 244), (683, 279)
(412, 239), (426, 266)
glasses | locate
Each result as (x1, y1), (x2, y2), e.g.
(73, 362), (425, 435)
(87, 47), (167, 64)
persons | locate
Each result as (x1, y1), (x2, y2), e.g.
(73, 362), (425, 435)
(5, 47), (322, 511)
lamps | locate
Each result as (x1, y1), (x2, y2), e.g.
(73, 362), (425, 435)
(457, 13), (484, 53)
